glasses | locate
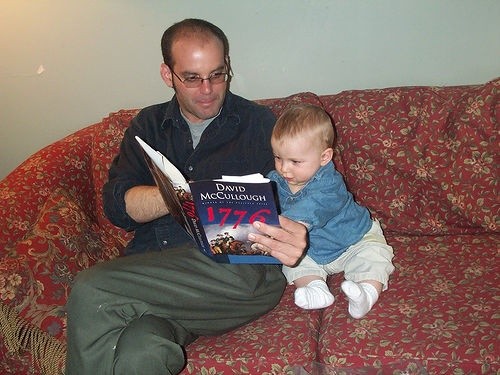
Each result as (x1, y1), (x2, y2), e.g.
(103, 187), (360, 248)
(168, 59), (234, 88)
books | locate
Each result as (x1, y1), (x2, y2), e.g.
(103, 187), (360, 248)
(134, 135), (284, 264)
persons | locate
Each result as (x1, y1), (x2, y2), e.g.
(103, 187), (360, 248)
(65, 18), (308, 375)
(264, 103), (395, 320)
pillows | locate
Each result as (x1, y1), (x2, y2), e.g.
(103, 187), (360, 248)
(0, 183), (127, 375)
(90, 90), (345, 236)
(330, 79), (500, 235)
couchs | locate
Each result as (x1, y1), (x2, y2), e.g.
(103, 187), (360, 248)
(0, 78), (500, 374)
